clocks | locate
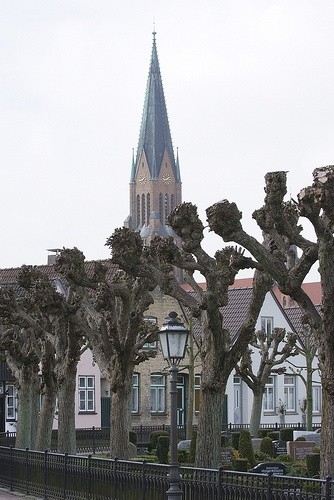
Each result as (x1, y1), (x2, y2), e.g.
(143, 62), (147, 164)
(161, 172), (173, 186)
(139, 169), (147, 185)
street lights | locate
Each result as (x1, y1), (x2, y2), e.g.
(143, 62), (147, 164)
(158, 312), (191, 500)
